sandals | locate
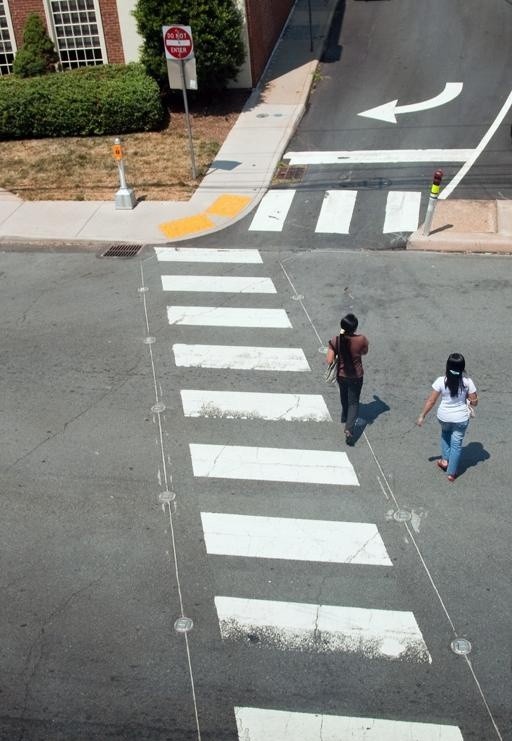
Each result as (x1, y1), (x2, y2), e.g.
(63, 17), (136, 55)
(437, 459), (455, 481)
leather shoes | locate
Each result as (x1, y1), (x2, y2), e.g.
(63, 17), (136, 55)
(346, 430), (352, 445)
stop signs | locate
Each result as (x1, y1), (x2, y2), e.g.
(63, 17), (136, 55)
(162, 25), (194, 60)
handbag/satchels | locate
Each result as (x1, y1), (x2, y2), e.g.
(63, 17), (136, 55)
(466, 395), (478, 418)
(322, 335), (338, 385)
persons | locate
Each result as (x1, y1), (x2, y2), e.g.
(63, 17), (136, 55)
(415, 353), (478, 480)
(326, 313), (369, 445)
